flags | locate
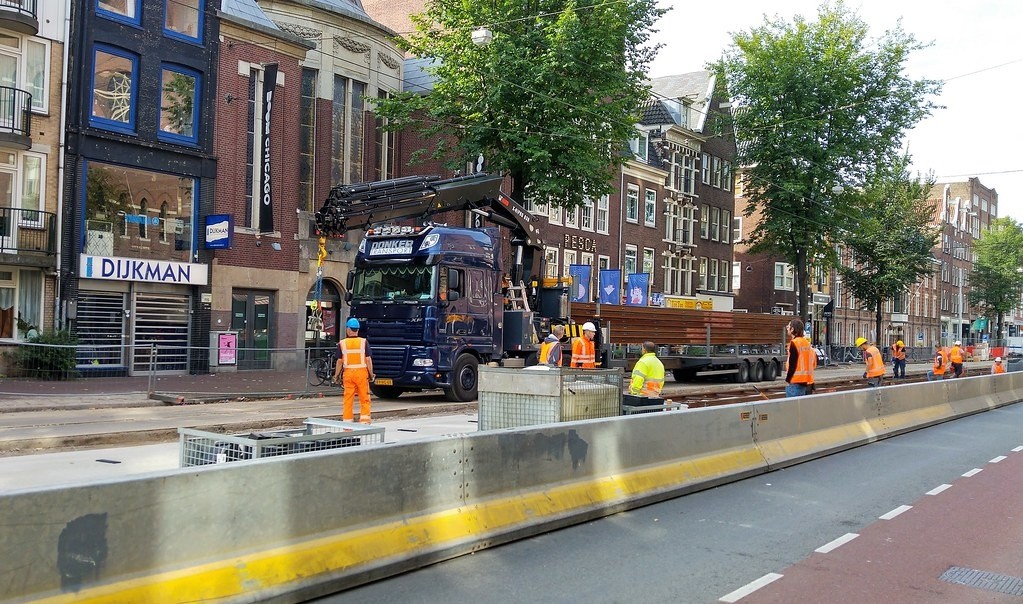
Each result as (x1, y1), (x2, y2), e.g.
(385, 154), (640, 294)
(821, 300), (833, 319)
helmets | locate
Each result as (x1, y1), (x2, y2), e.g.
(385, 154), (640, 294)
(346, 317), (360, 329)
(995, 356), (1001, 361)
(897, 341), (904, 347)
(955, 341), (962, 346)
(582, 322), (597, 332)
(856, 338), (867, 347)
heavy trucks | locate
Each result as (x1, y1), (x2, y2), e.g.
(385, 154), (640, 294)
(313, 170), (804, 403)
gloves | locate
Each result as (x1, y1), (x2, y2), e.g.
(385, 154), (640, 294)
(369, 374), (376, 382)
(333, 376), (340, 384)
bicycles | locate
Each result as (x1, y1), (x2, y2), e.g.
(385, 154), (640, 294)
(308, 342), (344, 389)
(729, 340), (919, 368)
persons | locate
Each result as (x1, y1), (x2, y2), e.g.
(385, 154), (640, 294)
(785, 319), (819, 398)
(628, 341), (666, 400)
(856, 338), (885, 387)
(991, 357), (1007, 374)
(536, 325), (565, 368)
(569, 322), (597, 368)
(947, 340), (971, 378)
(891, 340), (907, 380)
(927, 343), (949, 380)
(333, 318), (376, 434)
